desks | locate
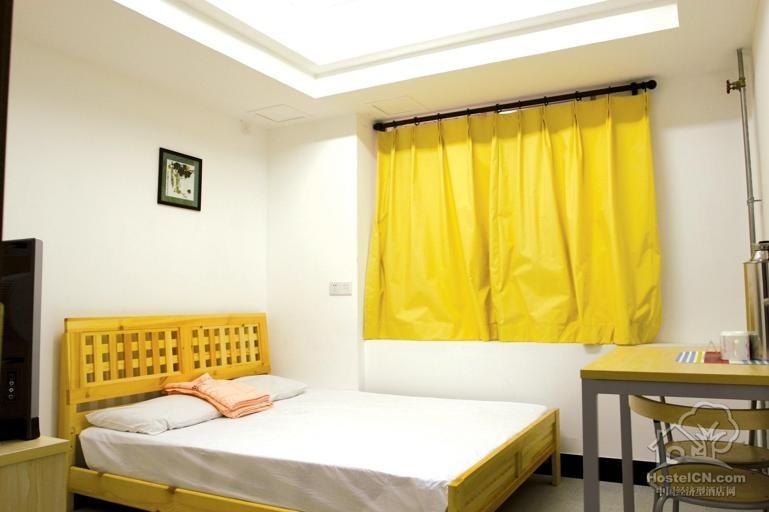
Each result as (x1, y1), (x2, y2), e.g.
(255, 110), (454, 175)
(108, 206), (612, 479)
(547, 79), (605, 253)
(578, 345), (769, 511)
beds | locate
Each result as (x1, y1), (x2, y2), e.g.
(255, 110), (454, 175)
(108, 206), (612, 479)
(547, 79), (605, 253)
(56, 310), (565, 512)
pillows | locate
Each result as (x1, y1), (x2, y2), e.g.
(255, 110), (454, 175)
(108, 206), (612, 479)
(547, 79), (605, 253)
(84, 370), (312, 436)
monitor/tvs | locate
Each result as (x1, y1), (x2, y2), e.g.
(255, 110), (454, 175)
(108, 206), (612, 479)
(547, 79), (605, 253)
(1, 238), (42, 442)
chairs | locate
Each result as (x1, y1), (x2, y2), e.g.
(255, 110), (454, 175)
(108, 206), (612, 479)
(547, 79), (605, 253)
(626, 393), (769, 511)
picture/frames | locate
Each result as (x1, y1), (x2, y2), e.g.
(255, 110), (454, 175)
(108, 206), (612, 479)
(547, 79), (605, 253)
(157, 147), (203, 210)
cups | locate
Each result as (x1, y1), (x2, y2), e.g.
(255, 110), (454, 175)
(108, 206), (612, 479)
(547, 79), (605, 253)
(719, 331), (751, 361)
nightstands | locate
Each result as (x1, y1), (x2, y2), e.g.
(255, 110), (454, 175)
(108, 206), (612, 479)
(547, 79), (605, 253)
(0, 438), (65, 512)
